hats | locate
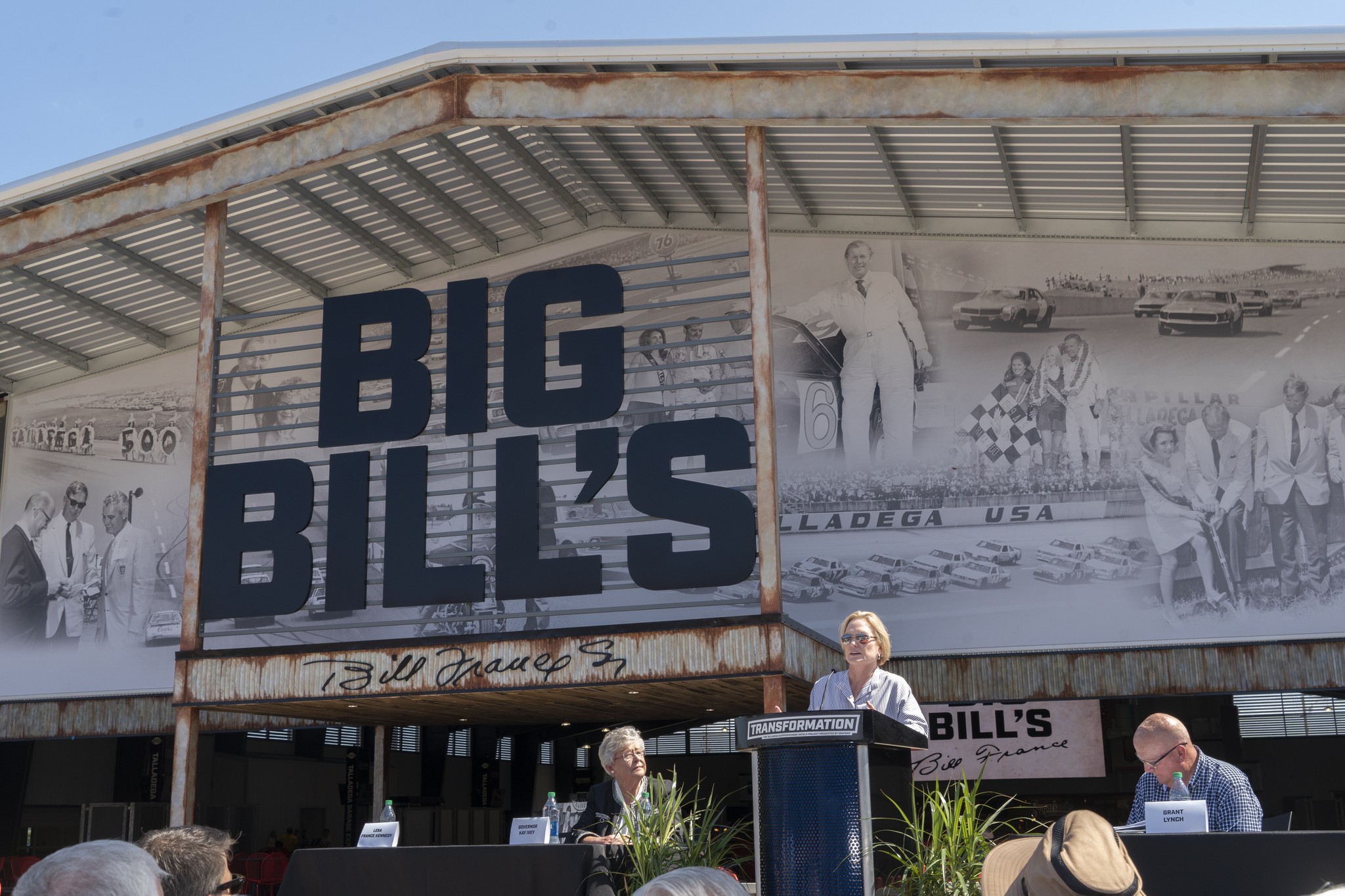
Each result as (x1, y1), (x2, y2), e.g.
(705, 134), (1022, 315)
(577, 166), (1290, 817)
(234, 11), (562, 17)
(980, 810), (1146, 896)
(724, 300), (748, 315)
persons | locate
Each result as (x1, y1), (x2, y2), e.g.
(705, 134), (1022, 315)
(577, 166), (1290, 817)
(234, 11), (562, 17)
(981, 809), (1147, 896)
(11, 413), (181, 465)
(633, 866), (751, 896)
(774, 610), (930, 738)
(569, 726), (684, 844)
(136, 825), (245, 896)
(11, 839), (171, 896)
(214, 301), (752, 635)
(0, 481), (156, 648)
(771, 239), (1345, 625)
(1127, 714), (1264, 832)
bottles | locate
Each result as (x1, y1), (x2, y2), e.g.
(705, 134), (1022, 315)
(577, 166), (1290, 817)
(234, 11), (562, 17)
(637, 792), (653, 843)
(380, 800), (396, 823)
(1169, 772), (1191, 801)
(542, 792), (560, 844)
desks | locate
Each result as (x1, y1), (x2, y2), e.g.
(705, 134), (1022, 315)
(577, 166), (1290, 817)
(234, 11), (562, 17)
(277, 843), (688, 895)
(1008, 831), (1345, 896)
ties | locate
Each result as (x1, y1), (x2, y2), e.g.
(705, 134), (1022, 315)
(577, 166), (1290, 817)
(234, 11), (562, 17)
(65, 522), (74, 576)
(1290, 413), (1301, 466)
(107, 538), (116, 586)
(1211, 438), (1221, 478)
(855, 279), (867, 300)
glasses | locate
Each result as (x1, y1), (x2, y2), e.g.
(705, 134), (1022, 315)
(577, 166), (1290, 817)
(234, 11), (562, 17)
(277, 401), (299, 410)
(41, 511), (52, 524)
(1138, 741), (1189, 769)
(66, 495), (87, 508)
(611, 750), (646, 762)
(212, 872), (246, 895)
(841, 633), (878, 646)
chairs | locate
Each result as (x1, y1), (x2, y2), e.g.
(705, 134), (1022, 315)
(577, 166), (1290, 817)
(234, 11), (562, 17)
(1261, 811), (1293, 831)
(0, 855), (42, 884)
(228, 847), (287, 896)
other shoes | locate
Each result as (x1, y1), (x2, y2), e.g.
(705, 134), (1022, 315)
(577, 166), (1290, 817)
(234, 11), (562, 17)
(1313, 587), (1333, 604)
(1279, 597), (1294, 611)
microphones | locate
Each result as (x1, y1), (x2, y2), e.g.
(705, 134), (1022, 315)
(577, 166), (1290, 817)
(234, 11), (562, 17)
(818, 663), (841, 711)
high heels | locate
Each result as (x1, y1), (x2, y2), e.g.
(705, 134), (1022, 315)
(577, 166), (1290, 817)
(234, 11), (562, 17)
(1207, 592), (1227, 610)
(1161, 608), (1182, 627)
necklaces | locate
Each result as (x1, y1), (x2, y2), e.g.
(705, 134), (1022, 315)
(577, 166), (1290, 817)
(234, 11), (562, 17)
(625, 798), (633, 803)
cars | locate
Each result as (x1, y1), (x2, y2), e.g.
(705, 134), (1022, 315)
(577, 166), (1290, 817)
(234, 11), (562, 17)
(1031, 534), (1149, 583)
(839, 539), (1024, 598)
(715, 554), (849, 604)
